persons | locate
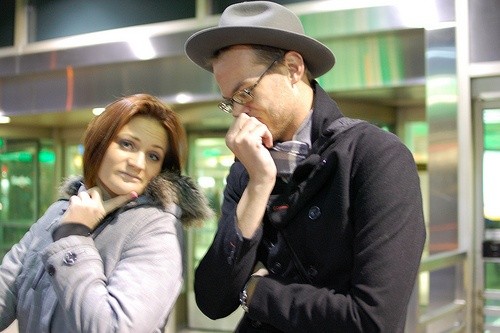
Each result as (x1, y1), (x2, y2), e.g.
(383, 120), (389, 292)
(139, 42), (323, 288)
(0, 93), (212, 333)
(184, 1), (427, 333)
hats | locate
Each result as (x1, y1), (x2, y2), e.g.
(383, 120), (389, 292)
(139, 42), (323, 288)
(183, 1), (337, 79)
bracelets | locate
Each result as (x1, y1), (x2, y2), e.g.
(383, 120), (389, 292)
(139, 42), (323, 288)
(239, 274), (263, 313)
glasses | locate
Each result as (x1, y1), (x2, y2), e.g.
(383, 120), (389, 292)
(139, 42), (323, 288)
(217, 56), (280, 114)
